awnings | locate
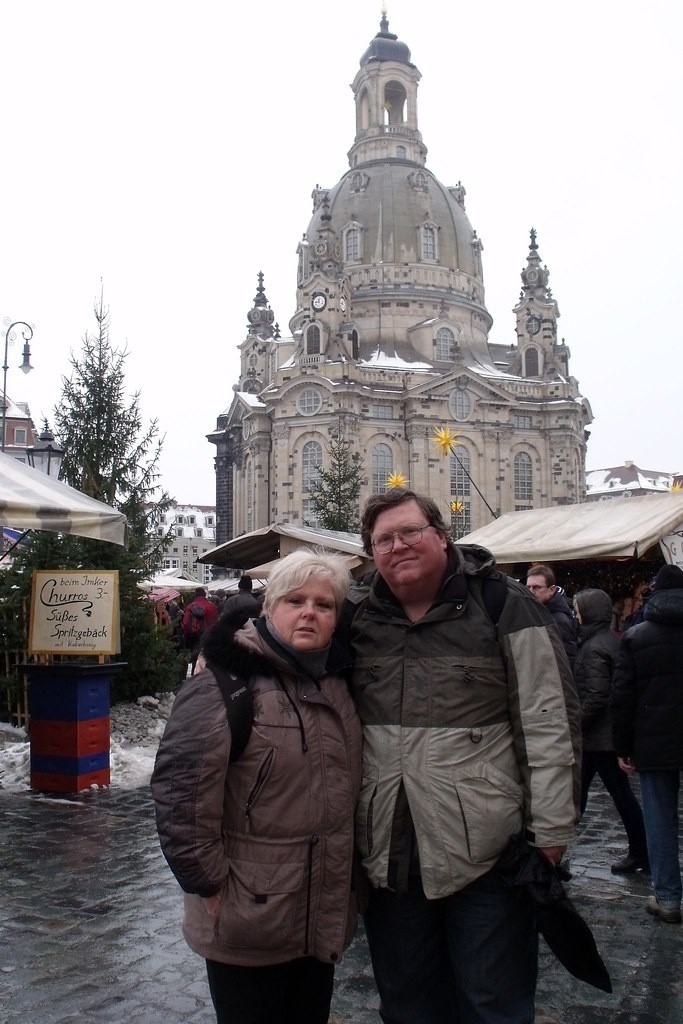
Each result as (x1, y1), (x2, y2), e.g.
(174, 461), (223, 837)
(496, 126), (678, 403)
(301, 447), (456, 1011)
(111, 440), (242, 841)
(0, 450), (129, 552)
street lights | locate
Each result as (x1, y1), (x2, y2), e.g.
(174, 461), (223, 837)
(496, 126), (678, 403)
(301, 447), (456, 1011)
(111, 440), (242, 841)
(26, 419), (66, 571)
(0, 316), (37, 454)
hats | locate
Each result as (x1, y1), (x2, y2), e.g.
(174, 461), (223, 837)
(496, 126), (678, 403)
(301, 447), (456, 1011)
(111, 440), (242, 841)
(237, 575), (252, 590)
(194, 587), (207, 598)
(215, 588), (227, 598)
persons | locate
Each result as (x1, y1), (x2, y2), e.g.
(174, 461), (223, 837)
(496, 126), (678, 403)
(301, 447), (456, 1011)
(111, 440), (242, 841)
(567, 588), (652, 877)
(344, 489), (585, 1024)
(610, 565), (683, 924)
(155, 581), (225, 659)
(522, 564), (575, 657)
(148, 548), (367, 1024)
(222, 572), (257, 620)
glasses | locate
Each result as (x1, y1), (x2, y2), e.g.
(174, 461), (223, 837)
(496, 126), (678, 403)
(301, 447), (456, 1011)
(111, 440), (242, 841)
(368, 523), (432, 555)
(525, 584), (547, 591)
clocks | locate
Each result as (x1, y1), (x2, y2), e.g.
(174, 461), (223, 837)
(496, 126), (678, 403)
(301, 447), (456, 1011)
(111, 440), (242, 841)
(340, 298), (346, 311)
(313, 296), (325, 308)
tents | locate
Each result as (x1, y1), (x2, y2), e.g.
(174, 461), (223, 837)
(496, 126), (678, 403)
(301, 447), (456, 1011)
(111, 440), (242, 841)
(144, 491), (682, 585)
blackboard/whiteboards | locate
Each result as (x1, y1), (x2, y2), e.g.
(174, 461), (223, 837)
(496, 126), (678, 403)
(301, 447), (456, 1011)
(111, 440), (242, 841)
(30, 568), (122, 656)
